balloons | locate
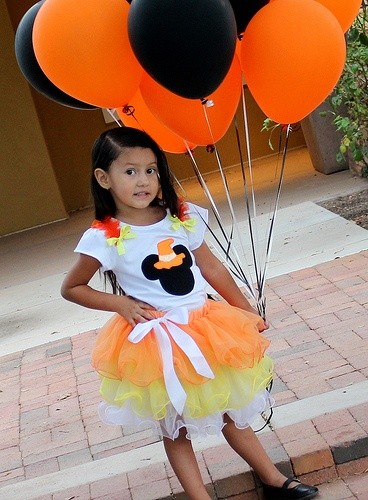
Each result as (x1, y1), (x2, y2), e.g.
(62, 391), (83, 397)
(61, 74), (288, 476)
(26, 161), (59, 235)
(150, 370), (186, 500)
(14, 0), (362, 154)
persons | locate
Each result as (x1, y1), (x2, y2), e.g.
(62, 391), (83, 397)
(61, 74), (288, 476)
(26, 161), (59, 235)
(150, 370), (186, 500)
(61, 127), (319, 500)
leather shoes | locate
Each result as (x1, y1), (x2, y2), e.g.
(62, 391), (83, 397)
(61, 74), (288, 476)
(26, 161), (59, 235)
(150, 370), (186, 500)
(262, 477), (318, 500)
